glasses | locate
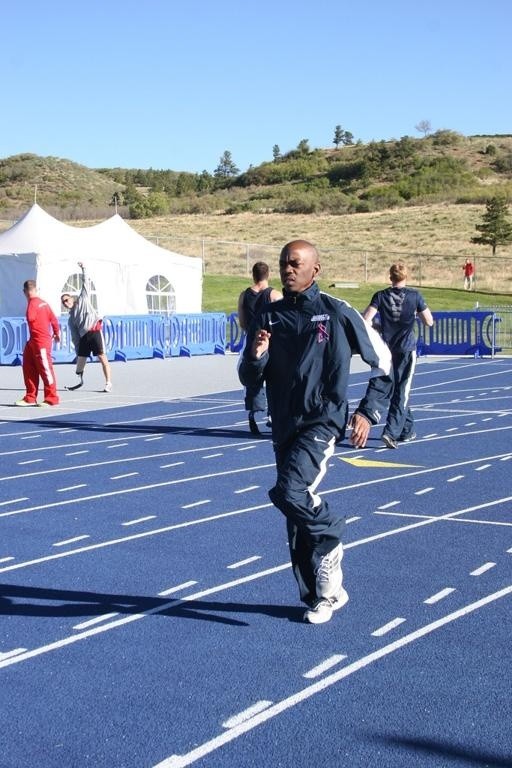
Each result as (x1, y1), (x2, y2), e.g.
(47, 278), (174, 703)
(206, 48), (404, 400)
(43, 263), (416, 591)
(62, 298), (71, 303)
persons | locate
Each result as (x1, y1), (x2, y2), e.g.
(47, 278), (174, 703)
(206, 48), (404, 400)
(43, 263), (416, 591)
(461, 258), (475, 292)
(236, 239), (397, 625)
(60, 259), (113, 394)
(14, 279), (61, 406)
(359, 263), (434, 449)
(238, 261), (283, 434)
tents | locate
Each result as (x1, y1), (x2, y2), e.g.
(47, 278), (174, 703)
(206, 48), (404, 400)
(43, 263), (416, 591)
(0, 184), (204, 365)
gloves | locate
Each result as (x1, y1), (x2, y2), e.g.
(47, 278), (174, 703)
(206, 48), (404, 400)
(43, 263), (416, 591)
(78, 262), (85, 269)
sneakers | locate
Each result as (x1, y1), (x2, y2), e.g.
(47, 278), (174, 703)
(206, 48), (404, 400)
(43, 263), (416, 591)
(303, 586), (349, 623)
(64, 375), (112, 392)
(381, 433), (398, 449)
(15, 399), (50, 406)
(316, 541), (343, 599)
(403, 431), (416, 441)
